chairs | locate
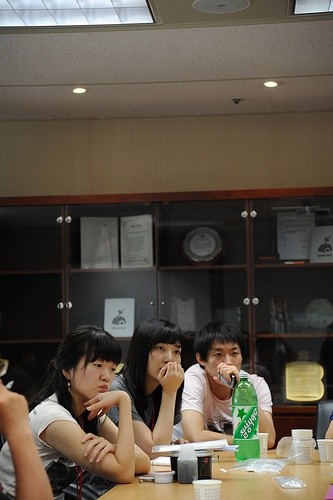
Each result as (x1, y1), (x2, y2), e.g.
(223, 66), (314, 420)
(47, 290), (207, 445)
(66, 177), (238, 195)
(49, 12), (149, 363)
(315, 399), (333, 449)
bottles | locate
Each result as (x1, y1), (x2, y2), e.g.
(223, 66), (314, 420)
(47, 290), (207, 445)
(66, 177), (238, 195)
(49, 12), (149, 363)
(231, 372), (260, 460)
(177, 445), (198, 484)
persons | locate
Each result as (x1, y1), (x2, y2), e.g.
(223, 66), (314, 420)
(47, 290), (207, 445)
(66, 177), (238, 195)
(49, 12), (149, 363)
(324, 408), (333, 439)
(0, 324), (151, 500)
(181, 318), (276, 450)
(0, 380), (55, 500)
(106, 317), (185, 459)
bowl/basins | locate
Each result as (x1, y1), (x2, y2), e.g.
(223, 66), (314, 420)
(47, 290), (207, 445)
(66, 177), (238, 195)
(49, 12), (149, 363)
(152, 471), (175, 482)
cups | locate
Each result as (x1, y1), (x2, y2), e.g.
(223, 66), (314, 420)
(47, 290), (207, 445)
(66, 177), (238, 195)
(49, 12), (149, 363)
(191, 480), (222, 500)
(258, 432), (269, 453)
(317, 439), (333, 462)
(292, 430), (314, 465)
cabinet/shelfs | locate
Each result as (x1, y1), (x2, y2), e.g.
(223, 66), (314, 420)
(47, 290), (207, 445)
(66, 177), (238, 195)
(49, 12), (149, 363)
(0, 185), (333, 451)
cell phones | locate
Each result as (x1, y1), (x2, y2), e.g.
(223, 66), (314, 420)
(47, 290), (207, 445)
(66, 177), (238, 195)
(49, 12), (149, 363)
(218, 372), (237, 388)
(139, 474), (155, 482)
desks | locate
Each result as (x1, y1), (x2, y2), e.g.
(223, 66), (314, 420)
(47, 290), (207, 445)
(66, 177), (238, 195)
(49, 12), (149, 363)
(95, 449), (333, 500)
